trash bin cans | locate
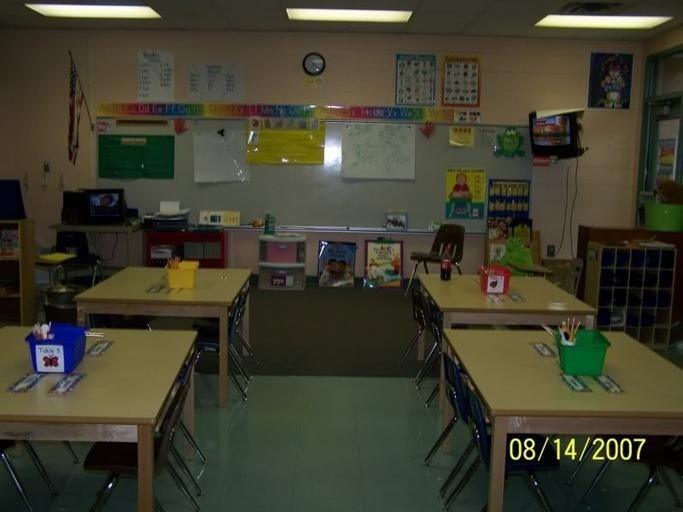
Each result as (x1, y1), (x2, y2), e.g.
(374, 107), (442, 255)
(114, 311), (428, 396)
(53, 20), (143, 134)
(41, 284), (88, 326)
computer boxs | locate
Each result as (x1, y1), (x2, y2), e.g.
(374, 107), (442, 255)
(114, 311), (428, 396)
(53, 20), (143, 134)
(63, 189), (84, 226)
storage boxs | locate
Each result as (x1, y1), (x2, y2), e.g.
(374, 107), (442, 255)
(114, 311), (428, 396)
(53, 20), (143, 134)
(256, 234), (306, 263)
(258, 263), (307, 293)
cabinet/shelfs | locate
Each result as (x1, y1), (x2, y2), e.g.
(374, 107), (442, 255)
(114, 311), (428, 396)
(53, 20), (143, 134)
(583, 242), (678, 352)
(141, 229), (228, 270)
(0, 218), (36, 326)
(486, 221), (539, 277)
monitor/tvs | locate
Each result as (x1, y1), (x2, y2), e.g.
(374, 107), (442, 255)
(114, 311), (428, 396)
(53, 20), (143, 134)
(84, 188), (124, 224)
(529, 111), (583, 158)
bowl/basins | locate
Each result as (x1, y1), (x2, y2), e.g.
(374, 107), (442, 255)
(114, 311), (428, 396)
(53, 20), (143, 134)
(644, 203), (682, 233)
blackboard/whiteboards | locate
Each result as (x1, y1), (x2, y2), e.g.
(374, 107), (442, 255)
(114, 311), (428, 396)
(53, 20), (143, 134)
(94, 116), (534, 235)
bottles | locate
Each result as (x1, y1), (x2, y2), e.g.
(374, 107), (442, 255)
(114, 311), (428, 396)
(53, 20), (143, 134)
(264, 213), (274, 235)
(440, 246), (451, 281)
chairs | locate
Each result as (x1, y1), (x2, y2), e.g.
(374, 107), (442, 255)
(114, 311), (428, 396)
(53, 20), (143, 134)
(405, 223), (467, 294)
(191, 274), (255, 401)
(404, 280), (498, 392)
(424, 351), (683, 510)
(3, 341), (206, 510)
(48, 229), (105, 288)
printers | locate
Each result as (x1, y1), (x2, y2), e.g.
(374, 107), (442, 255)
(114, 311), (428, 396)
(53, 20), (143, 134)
(144, 215), (187, 230)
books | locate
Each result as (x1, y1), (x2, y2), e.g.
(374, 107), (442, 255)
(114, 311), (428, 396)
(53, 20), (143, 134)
(154, 208), (193, 220)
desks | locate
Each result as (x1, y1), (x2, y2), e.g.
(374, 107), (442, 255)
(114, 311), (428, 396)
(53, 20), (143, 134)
(414, 272), (599, 418)
(1, 326), (197, 511)
(442, 330), (683, 512)
(49, 223), (146, 265)
(74, 266), (252, 409)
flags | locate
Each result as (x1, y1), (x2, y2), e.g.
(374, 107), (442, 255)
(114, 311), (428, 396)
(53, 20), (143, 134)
(66, 53), (85, 168)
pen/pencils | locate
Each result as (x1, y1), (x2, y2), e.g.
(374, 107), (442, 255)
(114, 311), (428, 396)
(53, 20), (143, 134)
(33, 320), (54, 340)
(561, 317), (581, 342)
(167, 255), (181, 269)
(481, 265), (495, 276)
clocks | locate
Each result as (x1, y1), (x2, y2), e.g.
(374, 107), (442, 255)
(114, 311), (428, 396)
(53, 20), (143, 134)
(302, 51), (325, 75)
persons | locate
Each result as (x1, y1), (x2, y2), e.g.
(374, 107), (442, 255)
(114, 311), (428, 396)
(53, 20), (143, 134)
(449, 173), (474, 213)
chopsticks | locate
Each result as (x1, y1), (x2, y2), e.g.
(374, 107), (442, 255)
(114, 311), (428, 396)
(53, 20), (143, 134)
(539, 317), (580, 340)
(165, 255), (181, 270)
